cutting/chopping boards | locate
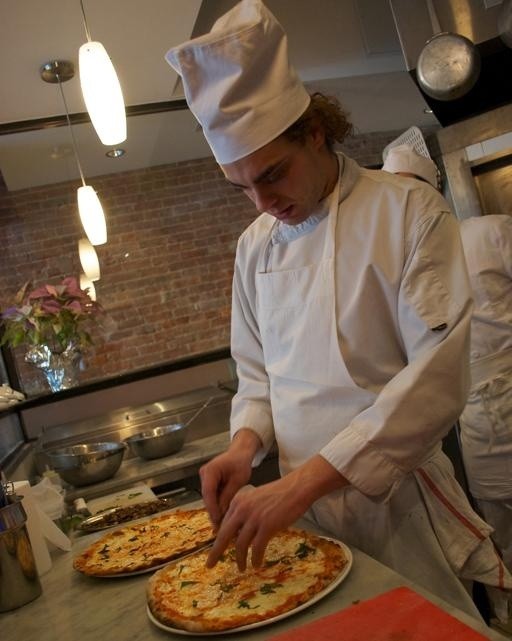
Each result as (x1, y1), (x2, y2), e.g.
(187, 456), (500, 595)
(96, 508), (213, 581)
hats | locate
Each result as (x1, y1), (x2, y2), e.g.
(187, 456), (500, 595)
(382, 144), (438, 191)
(162, 0), (311, 169)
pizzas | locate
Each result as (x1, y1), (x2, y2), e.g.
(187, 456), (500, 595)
(72, 508), (216, 579)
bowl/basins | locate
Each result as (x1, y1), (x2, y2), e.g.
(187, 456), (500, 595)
(45, 439), (127, 486)
(123, 422), (190, 457)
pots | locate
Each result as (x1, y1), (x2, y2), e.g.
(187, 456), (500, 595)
(415, 30), (483, 101)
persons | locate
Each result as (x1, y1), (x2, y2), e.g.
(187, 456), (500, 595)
(379, 141), (511, 625)
(164, 0), (512, 629)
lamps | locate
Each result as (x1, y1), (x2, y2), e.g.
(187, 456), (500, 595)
(79, 270), (97, 305)
(78, 1), (128, 145)
(40, 59), (108, 247)
(73, 238), (102, 283)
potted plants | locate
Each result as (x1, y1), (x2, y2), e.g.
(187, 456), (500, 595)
(6, 271), (100, 393)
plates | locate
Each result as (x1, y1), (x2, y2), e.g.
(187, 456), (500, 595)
(75, 503), (161, 530)
